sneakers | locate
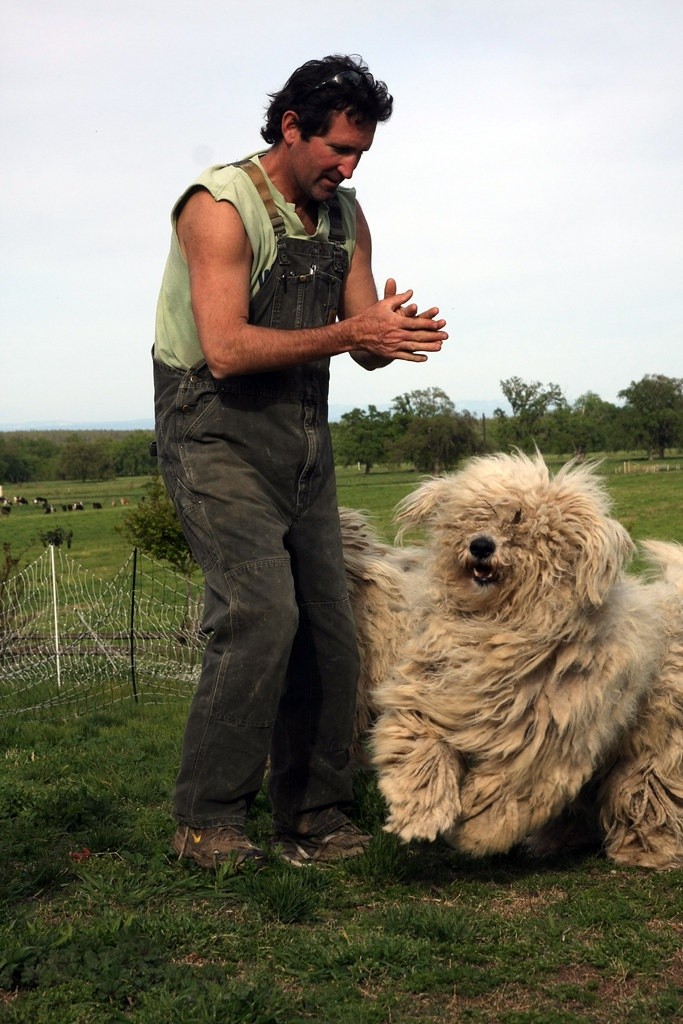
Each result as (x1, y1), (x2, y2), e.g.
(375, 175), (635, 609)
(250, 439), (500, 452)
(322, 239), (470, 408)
(175, 824), (266, 870)
(273, 812), (373, 867)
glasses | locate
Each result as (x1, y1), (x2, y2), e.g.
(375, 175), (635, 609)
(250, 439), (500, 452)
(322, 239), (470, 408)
(313, 72), (375, 93)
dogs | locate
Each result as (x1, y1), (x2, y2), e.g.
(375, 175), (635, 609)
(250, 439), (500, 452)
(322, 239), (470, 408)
(339, 433), (682, 870)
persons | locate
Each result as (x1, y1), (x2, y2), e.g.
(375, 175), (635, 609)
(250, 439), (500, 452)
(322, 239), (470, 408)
(151, 55), (450, 872)
